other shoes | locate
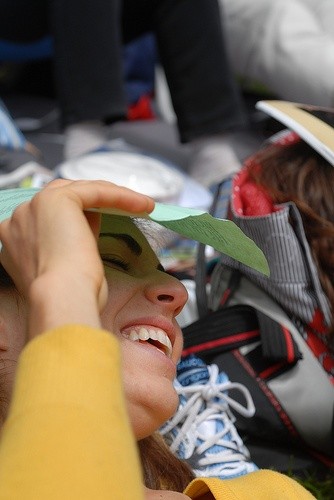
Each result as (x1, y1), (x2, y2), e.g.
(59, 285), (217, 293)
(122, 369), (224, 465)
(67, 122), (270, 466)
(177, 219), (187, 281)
(63, 121), (107, 161)
(183, 140), (238, 184)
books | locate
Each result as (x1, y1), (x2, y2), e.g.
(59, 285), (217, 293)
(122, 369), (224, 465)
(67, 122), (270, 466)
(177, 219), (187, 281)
(255, 98), (334, 166)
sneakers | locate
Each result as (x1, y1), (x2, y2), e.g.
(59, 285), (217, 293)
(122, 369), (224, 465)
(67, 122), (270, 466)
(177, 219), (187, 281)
(158, 355), (259, 479)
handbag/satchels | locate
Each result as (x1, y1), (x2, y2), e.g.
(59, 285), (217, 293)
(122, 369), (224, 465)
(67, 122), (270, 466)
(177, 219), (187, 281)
(174, 125), (334, 470)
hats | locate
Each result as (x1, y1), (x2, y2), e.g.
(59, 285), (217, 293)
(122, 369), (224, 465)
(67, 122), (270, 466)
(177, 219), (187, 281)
(0, 101), (50, 187)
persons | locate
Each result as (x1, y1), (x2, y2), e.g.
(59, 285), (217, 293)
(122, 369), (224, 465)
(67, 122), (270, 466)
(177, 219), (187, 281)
(0, 178), (317, 500)
(249, 138), (334, 306)
(0, 0), (261, 478)
